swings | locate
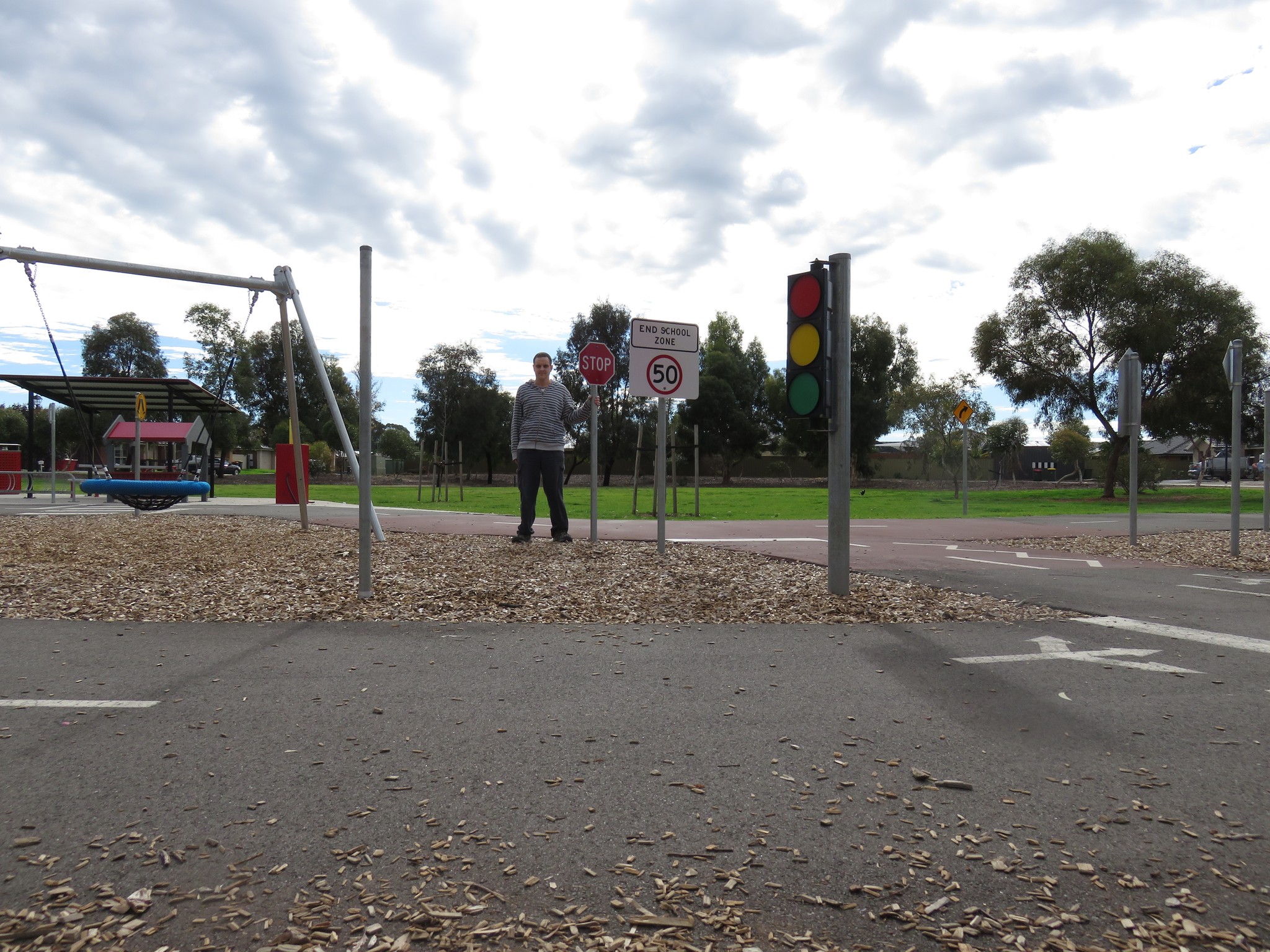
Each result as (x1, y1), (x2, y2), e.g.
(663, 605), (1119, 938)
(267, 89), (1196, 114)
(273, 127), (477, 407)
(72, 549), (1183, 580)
(16, 258), (262, 515)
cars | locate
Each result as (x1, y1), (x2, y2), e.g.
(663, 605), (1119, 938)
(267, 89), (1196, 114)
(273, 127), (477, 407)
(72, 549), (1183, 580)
(175, 455), (241, 476)
(1188, 460), (1212, 479)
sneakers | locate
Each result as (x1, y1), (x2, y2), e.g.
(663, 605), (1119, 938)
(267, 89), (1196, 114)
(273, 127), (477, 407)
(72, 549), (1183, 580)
(553, 532), (573, 542)
(511, 534), (531, 543)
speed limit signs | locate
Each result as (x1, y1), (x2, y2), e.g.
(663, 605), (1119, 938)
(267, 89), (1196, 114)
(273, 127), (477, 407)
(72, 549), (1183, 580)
(647, 354), (683, 395)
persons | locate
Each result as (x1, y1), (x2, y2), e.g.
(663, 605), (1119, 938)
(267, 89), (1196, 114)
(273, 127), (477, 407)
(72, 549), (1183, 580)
(511, 352), (600, 543)
(1252, 462), (1264, 481)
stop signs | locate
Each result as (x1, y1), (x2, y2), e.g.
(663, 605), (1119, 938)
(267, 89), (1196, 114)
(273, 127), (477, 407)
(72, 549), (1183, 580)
(578, 341), (614, 385)
